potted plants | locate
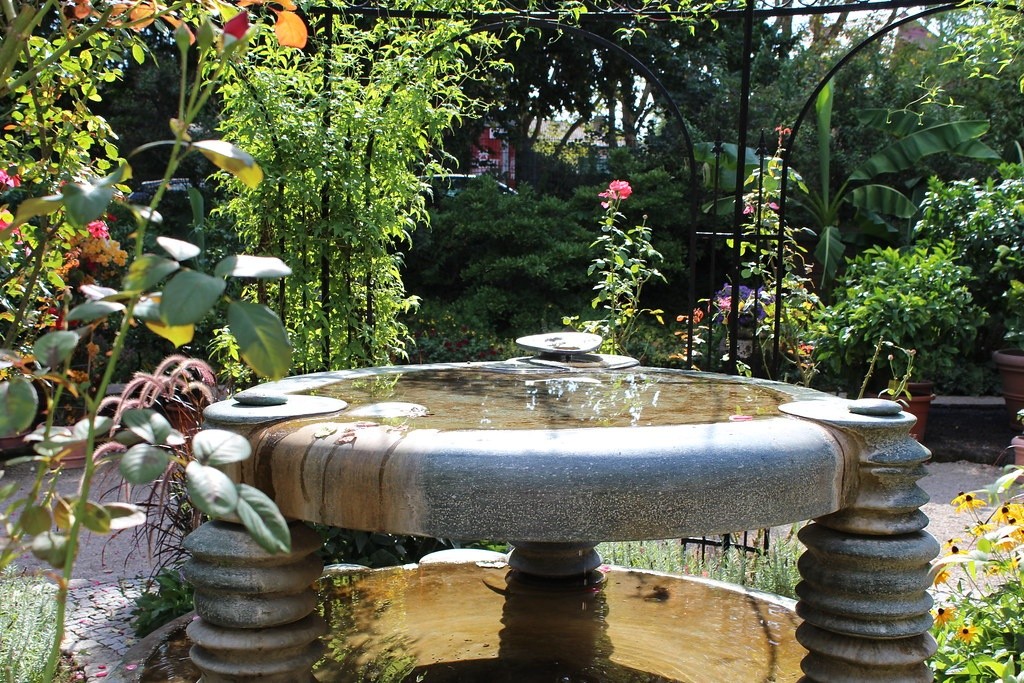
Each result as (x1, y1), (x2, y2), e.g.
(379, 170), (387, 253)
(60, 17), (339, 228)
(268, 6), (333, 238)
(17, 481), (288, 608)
(806, 274), (968, 438)
(1011, 409), (1024, 484)
(148, 345), (219, 454)
(822, 238), (990, 391)
(913, 162), (1024, 435)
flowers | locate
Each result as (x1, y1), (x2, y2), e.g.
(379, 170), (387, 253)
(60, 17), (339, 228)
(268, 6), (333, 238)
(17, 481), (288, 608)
(40, 342), (105, 425)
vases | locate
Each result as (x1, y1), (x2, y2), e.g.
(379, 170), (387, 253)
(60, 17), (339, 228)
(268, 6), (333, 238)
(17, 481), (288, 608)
(37, 420), (87, 470)
(0, 430), (33, 449)
